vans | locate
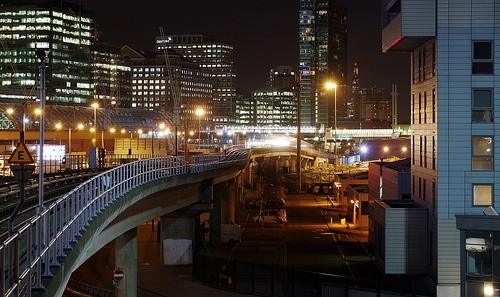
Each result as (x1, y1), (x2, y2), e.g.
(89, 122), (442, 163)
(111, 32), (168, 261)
(204, 222), (242, 247)
(254, 209), (287, 224)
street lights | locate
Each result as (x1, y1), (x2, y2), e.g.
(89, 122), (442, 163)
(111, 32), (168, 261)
(35, 50), (45, 216)
(194, 108), (206, 149)
(90, 103), (98, 149)
(324, 81), (338, 172)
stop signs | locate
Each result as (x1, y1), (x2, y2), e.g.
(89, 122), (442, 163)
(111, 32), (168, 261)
(113, 270), (125, 282)
